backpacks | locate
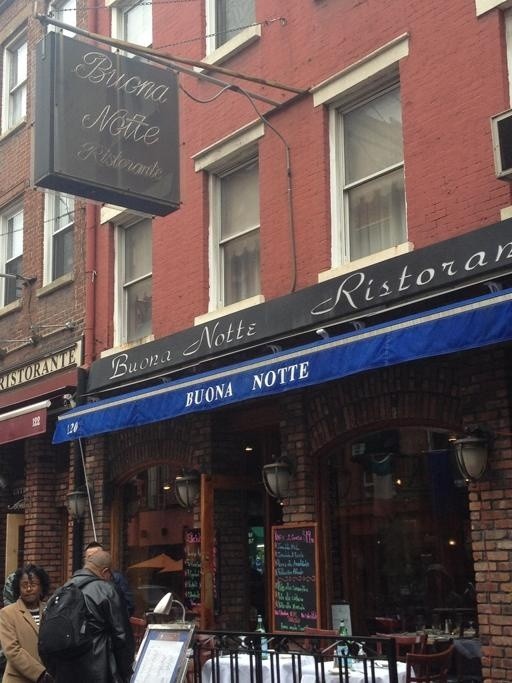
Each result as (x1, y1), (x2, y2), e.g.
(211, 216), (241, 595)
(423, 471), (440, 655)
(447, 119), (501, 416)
(38, 576), (105, 663)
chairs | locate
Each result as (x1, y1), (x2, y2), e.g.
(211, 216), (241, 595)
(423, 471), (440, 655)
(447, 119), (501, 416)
(303, 625), (456, 683)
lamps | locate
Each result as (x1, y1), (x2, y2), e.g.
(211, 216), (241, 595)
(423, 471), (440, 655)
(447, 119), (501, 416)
(171, 466), (200, 512)
(154, 592), (186, 624)
(65, 484), (95, 523)
(262, 452), (297, 505)
(452, 422), (490, 481)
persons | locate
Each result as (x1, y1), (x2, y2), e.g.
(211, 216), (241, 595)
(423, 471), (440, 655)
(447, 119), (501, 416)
(0, 562), (55, 683)
(38, 550), (136, 683)
(85, 541), (135, 618)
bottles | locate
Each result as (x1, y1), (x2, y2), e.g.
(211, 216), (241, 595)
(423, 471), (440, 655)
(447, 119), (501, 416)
(336, 618), (353, 669)
(255, 614), (268, 660)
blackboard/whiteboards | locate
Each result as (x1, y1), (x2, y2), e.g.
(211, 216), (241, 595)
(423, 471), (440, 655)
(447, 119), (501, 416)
(182, 527), (221, 616)
(272, 522), (321, 635)
